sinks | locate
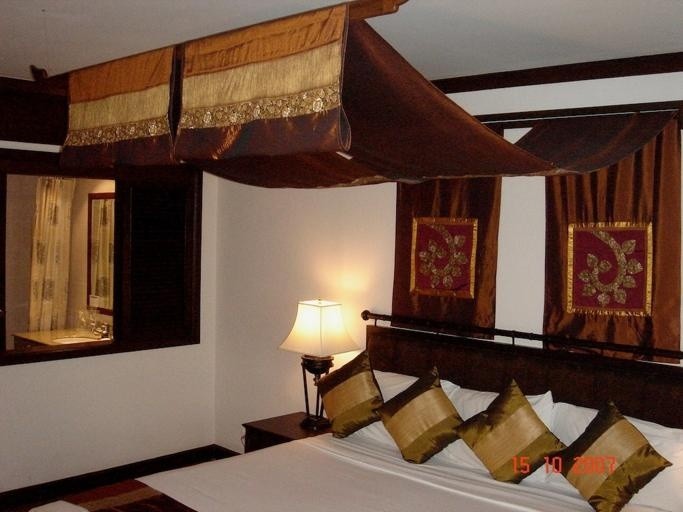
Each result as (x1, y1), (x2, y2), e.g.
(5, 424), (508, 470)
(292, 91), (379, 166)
(53, 335), (104, 345)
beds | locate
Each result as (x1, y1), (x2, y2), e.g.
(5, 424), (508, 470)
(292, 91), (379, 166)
(26, 310), (683, 511)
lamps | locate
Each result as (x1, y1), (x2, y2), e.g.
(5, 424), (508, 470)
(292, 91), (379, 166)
(279, 298), (361, 433)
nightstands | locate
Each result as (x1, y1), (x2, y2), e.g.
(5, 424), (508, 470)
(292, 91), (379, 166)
(241, 412), (332, 454)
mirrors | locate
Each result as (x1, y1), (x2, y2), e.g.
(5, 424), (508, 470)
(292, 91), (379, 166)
(86, 192), (116, 316)
(0, 165), (121, 364)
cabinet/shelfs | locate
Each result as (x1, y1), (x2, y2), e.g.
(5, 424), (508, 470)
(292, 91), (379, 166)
(14, 336), (47, 351)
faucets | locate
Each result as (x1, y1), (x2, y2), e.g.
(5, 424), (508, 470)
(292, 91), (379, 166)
(94, 323), (113, 338)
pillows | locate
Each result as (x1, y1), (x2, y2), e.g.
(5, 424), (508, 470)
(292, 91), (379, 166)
(315, 346), (385, 439)
(433, 386), (554, 486)
(372, 360), (465, 465)
(452, 375), (569, 485)
(346, 367), (461, 451)
